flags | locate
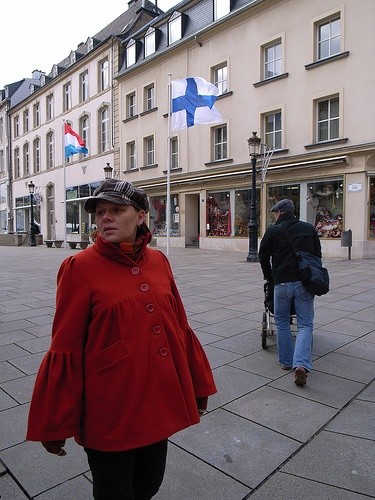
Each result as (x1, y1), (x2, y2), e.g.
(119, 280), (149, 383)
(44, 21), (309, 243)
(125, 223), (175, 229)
(63, 123), (89, 158)
(171, 77), (221, 132)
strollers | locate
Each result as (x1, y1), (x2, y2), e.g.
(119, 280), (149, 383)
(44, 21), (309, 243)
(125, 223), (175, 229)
(259, 279), (296, 350)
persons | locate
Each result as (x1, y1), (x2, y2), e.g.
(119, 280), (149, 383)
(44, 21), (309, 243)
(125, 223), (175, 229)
(259, 199), (321, 385)
(26, 177), (217, 500)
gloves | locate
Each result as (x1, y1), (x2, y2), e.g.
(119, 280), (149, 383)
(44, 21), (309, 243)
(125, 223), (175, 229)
(195, 397), (210, 416)
(42, 440), (67, 456)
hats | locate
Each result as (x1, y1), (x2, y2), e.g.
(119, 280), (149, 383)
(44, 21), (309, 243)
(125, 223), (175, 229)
(271, 199), (294, 213)
(84, 179), (149, 217)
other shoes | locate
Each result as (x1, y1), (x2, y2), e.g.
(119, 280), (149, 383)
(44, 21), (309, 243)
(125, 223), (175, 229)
(278, 359), (292, 369)
(293, 368), (307, 387)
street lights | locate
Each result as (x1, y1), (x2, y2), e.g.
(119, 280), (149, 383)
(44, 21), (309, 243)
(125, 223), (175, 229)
(27, 180), (36, 246)
(246, 132), (262, 263)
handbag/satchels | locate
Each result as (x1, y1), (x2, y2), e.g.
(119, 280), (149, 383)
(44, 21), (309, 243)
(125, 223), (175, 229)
(295, 251), (330, 296)
